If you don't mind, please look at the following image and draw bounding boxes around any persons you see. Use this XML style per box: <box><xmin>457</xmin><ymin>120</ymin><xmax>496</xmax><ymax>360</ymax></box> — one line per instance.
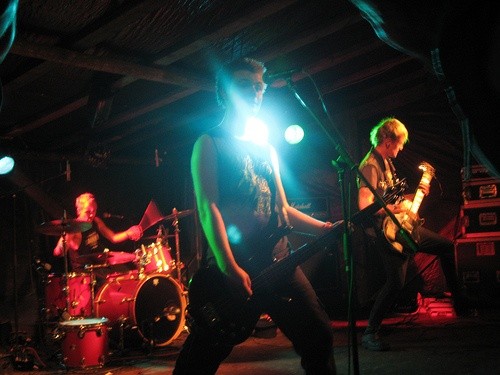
<box><xmin>357</xmin><ymin>119</ymin><xmax>477</xmax><ymax>351</ymax></box>
<box><xmin>54</xmin><ymin>193</ymin><xmax>143</xmax><ymax>294</ymax></box>
<box><xmin>172</xmin><ymin>58</ymin><xmax>352</xmax><ymax>375</ymax></box>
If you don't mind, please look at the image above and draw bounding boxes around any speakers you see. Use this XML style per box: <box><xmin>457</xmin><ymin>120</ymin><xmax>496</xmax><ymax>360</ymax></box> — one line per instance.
<box><xmin>453</xmin><ymin>239</ymin><xmax>500</xmax><ymax>319</ymax></box>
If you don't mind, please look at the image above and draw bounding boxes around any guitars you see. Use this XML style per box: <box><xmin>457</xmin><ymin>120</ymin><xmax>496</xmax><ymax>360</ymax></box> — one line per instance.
<box><xmin>186</xmin><ymin>172</ymin><xmax>407</xmax><ymax>346</ymax></box>
<box><xmin>382</xmin><ymin>160</ymin><xmax>437</xmax><ymax>257</ymax></box>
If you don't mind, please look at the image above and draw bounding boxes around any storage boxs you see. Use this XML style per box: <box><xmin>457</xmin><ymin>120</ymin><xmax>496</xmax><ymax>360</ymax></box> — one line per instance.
<box><xmin>454</xmin><ymin>165</ymin><xmax>500</xmax><ymax>317</ymax></box>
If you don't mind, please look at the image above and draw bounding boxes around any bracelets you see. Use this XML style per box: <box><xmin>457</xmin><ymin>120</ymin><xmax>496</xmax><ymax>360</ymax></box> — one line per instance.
<box><xmin>321</xmin><ymin>222</ymin><xmax>331</xmax><ymax>234</ymax></box>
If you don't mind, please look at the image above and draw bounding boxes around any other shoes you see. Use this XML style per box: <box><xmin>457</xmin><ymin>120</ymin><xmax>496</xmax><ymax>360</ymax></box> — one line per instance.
<box><xmin>362</xmin><ymin>327</ymin><xmax>390</xmax><ymax>351</ymax></box>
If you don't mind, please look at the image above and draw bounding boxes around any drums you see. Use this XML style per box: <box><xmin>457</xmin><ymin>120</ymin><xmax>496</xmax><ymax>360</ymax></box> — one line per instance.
<box><xmin>57</xmin><ymin>316</ymin><xmax>111</xmax><ymax>371</ymax></box>
<box><xmin>43</xmin><ymin>272</ymin><xmax>97</xmax><ymax>322</ymax></box>
<box><xmin>93</xmin><ymin>272</ymin><xmax>188</xmax><ymax>349</ymax></box>
<box><xmin>132</xmin><ymin>242</ymin><xmax>178</xmax><ymax>274</ymax></box>
<box><xmin>133</xmin><ymin>241</ymin><xmax>178</xmax><ymax>278</ymax></box>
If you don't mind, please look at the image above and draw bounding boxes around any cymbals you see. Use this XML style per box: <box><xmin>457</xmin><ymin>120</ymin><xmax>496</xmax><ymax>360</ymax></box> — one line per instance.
<box><xmin>34</xmin><ymin>218</ymin><xmax>93</xmax><ymax>236</ymax></box>
<box><xmin>139</xmin><ymin>234</ymin><xmax>176</xmax><ymax>240</ymax></box>
<box><xmin>69</xmin><ymin>251</ymin><xmax>136</xmax><ymax>266</ymax></box>
<box><xmin>159</xmin><ymin>208</ymin><xmax>195</xmax><ymax>220</ymax></box>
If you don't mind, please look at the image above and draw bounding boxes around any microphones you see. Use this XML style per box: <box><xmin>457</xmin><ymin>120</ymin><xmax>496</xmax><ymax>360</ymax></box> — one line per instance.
<box><xmin>263</xmin><ymin>67</ymin><xmax>302</xmax><ymax>83</ymax></box>
